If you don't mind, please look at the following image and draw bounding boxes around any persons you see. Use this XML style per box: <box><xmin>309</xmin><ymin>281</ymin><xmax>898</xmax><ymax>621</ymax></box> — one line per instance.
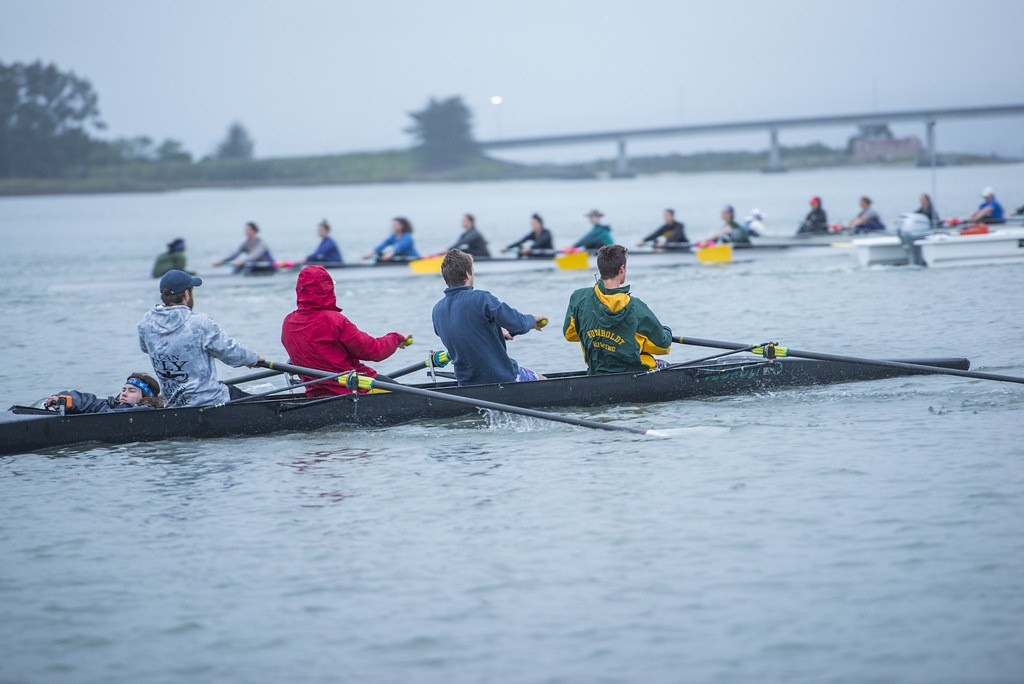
<box><xmin>712</xmin><ymin>206</ymin><xmax>750</xmax><ymax>242</ymax></box>
<box><xmin>432</xmin><ymin>248</ymin><xmax>547</xmax><ymax>386</ymax></box>
<box><xmin>971</xmin><ymin>189</ymin><xmax>1005</xmax><ymax>223</ymax></box>
<box><xmin>213</xmin><ymin>223</ymin><xmax>277</xmax><ymax>273</ymax></box>
<box><xmin>44</xmin><ymin>373</ymin><xmax>160</xmax><ymax>414</ymax></box>
<box><xmin>138</xmin><ymin>270</ymin><xmax>266</xmax><ymax>408</ymax></box>
<box><xmin>744</xmin><ymin>209</ymin><xmax>775</xmax><ymax>237</ymax></box>
<box><xmin>848</xmin><ymin>197</ymin><xmax>886</xmax><ymax>230</ymax></box>
<box><xmin>303</xmin><ymin>222</ymin><xmax>342</xmax><ymax>262</ymax></box>
<box><xmin>281</xmin><ymin>265</ymin><xmax>412</xmax><ymax>397</ymax></box>
<box><xmin>640</xmin><ymin>209</ymin><xmax>690</xmax><ymax>250</ymax></box>
<box><xmin>563</xmin><ymin>244</ymin><xmax>672</xmax><ymax>376</ymax></box>
<box><xmin>917</xmin><ymin>194</ymin><xmax>939</xmax><ymax>220</ymax></box>
<box><xmin>448</xmin><ymin>214</ymin><xmax>489</xmax><ymax>256</ymax></box>
<box><xmin>152</xmin><ymin>239</ymin><xmax>187</xmax><ymax>279</ymax></box>
<box><xmin>362</xmin><ymin>217</ymin><xmax>419</xmax><ymax>262</ymax></box>
<box><xmin>574</xmin><ymin>210</ymin><xmax>613</xmax><ymax>248</ymax></box>
<box><xmin>799</xmin><ymin>198</ymin><xmax>827</xmax><ymax>232</ymax></box>
<box><xmin>501</xmin><ymin>214</ymin><xmax>556</xmax><ymax>257</ymax></box>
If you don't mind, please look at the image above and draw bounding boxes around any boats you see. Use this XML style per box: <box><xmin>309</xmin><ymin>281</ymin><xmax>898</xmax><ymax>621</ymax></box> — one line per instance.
<box><xmin>0</xmin><ymin>345</ymin><xmax>972</xmax><ymax>461</ymax></box>
<box><xmin>44</xmin><ymin>211</ymin><xmax>1024</xmax><ymax>299</ymax></box>
<box><xmin>828</xmin><ymin>219</ymin><xmax>1024</xmax><ymax>272</ymax></box>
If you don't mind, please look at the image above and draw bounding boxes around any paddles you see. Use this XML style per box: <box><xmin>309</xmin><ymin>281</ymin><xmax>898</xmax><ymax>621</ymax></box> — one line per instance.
<box><xmin>389</xmin><ymin>317</ymin><xmax>547</xmax><ymax>380</ymax></box>
<box><xmin>224</xmin><ymin>253</ymin><xmax>445</xmax><ymax>276</ymax></box>
<box><xmin>223</xmin><ymin>337</ymin><xmax>413</xmax><ymax>385</ymax></box>
<box><xmin>671</xmin><ymin>334</ymin><xmax>1024</xmax><ymax>385</ymax></box>
<box><xmin>257</xmin><ymin>358</ymin><xmax>730</xmax><ymax>440</ymax></box>
<box><xmin>364</xmin><ymin>251</ymin><xmax>590</xmax><ymax>271</ymax></box>
<box><xmin>521</xmin><ymin>243</ymin><xmax>734</xmax><ymax>264</ymax></box>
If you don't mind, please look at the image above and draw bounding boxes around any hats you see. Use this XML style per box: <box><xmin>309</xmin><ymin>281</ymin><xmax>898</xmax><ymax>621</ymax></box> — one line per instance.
<box><xmin>977</xmin><ymin>187</ymin><xmax>994</xmax><ymax>197</ymax></box>
<box><xmin>583</xmin><ymin>209</ymin><xmax>604</xmax><ymax>217</ymax></box>
<box><xmin>160</xmin><ymin>270</ymin><xmax>202</xmax><ymax>295</ymax></box>
